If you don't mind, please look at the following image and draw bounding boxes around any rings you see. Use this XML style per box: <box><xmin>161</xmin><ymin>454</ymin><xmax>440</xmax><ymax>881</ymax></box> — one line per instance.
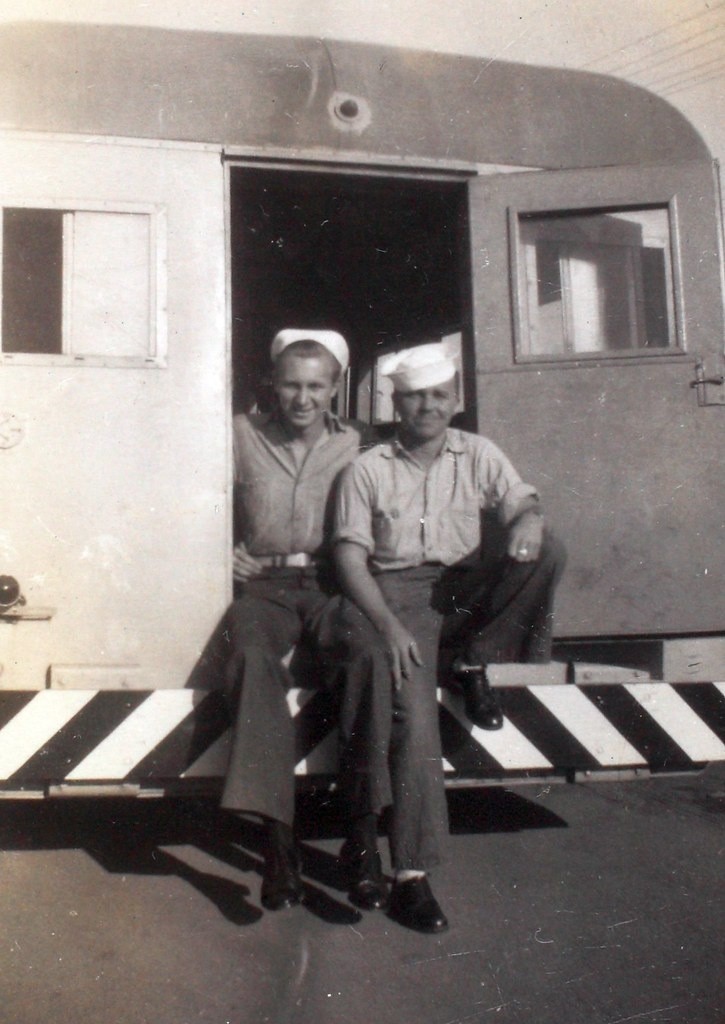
<box><xmin>516</xmin><ymin>548</ymin><xmax>528</xmax><ymax>557</ymax></box>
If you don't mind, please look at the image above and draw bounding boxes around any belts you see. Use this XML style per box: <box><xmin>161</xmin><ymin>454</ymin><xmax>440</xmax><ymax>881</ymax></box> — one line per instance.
<box><xmin>256</xmin><ymin>553</ymin><xmax>323</xmax><ymax>568</ymax></box>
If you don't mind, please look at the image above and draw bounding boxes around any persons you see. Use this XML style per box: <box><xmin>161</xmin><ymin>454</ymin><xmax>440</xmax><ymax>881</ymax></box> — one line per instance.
<box><xmin>330</xmin><ymin>341</ymin><xmax>566</xmax><ymax>933</ymax></box>
<box><xmin>210</xmin><ymin>325</ymin><xmax>397</xmax><ymax>911</ymax></box>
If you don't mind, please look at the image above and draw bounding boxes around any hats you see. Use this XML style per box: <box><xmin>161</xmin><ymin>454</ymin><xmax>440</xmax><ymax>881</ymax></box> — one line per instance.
<box><xmin>378</xmin><ymin>342</ymin><xmax>456</xmax><ymax>392</ymax></box>
<box><xmin>270</xmin><ymin>329</ymin><xmax>350</xmax><ymax>372</ymax></box>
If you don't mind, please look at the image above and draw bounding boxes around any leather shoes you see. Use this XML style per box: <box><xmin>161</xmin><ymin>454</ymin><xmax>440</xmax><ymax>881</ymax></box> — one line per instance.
<box><xmin>443</xmin><ymin>660</ymin><xmax>504</xmax><ymax>730</ymax></box>
<box><xmin>261</xmin><ymin>840</ymin><xmax>303</xmax><ymax>909</ymax></box>
<box><xmin>336</xmin><ymin>843</ymin><xmax>387</xmax><ymax>910</ymax></box>
<box><xmin>385</xmin><ymin>873</ymin><xmax>448</xmax><ymax>933</ymax></box>
